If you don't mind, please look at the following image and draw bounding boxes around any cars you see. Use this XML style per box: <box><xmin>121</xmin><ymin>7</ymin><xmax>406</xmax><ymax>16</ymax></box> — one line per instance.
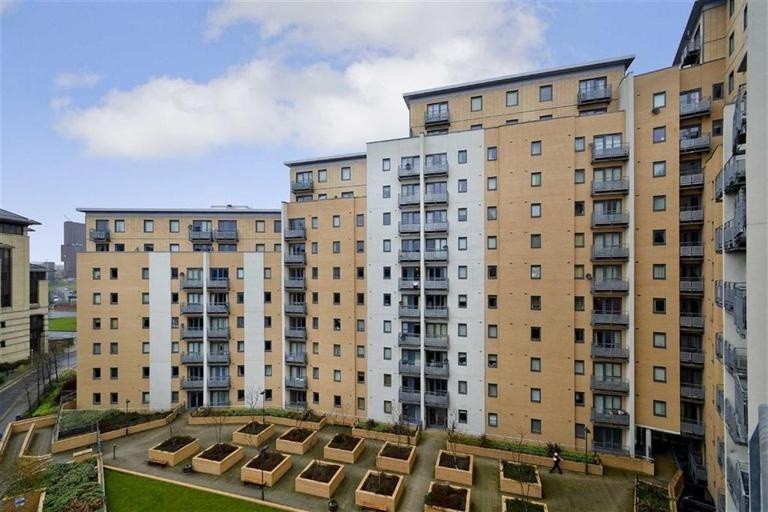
<box><xmin>53</xmin><ymin>289</ymin><xmax>76</xmax><ymax>304</ymax></box>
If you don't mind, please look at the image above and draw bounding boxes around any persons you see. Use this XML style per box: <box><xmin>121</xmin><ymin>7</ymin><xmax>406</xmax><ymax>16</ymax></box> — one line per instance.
<box><xmin>549</xmin><ymin>449</ymin><xmax>563</xmax><ymax>474</ymax></box>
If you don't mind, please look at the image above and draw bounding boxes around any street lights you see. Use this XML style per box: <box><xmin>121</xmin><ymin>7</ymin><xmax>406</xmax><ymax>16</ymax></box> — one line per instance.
<box><xmin>584</xmin><ymin>426</ymin><xmax>591</xmax><ymax>473</ymax></box>
<box><xmin>260</xmin><ymin>445</ymin><xmax>270</xmax><ymax>500</ymax></box>
<box><xmin>260</xmin><ymin>391</ymin><xmax>265</xmax><ymax>423</ymax></box>
<box><xmin>126</xmin><ymin>398</ymin><xmax>131</xmax><ymax>436</ymax></box>
